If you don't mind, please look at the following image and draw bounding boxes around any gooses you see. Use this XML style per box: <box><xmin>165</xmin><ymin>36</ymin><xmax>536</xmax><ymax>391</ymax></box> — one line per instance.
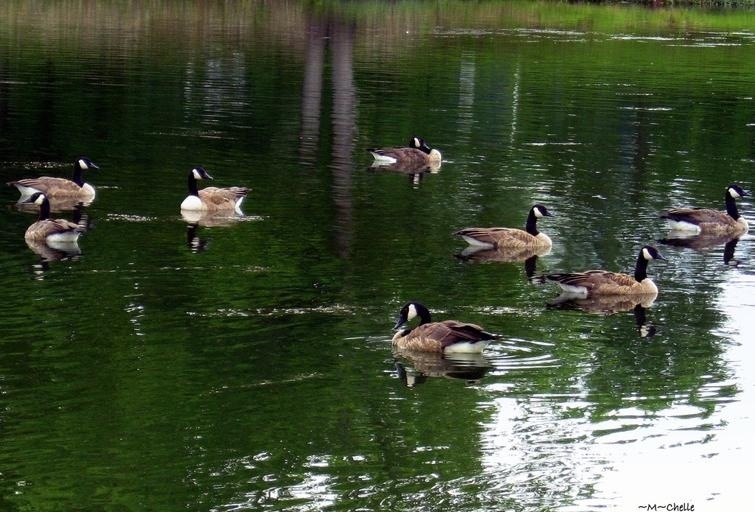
<box><xmin>550</xmin><ymin>247</ymin><xmax>670</xmax><ymax>297</ymax></box>
<box><xmin>451</xmin><ymin>203</ymin><xmax>557</xmax><ymax>249</ymax></box>
<box><xmin>392</xmin><ymin>347</ymin><xmax>496</xmax><ymax>388</ymax></box>
<box><xmin>546</xmin><ymin>291</ymin><xmax>660</xmax><ymax>340</ymax></box>
<box><xmin>383</xmin><ymin>303</ymin><xmax>504</xmax><ymax>356</ymax></box>
<box><xmin>6</xmin><ymin>157</ymin><xmax>99</xmax><ymax>243</ymax></box>
<box><xmin>659</xmin><ymin>185</ymin><xmax>753</xmax><ymax>232</ymax></box>
<box><xmin>454</xmin><ymin>244</ymin><xmax>552</xmax><ymax>288</ymax></box>
<box><xmin>180</xmin><ymin>209</ymin><xmax>264</xmax><ymax>251</ymax></box>
<box><xmin>180</xmin><ymin>167</ymin><xmax>253</xmax><ymax>209</ymax></box>
<box><xmin>365</xmin><ymin>136</ymin><xmax>441</xmax><ymax>163</ymax></box>
<box><xmin>655</xmin><ymin>230</ymin><xmax>747</xmax><ymax>268</ymax></box>
